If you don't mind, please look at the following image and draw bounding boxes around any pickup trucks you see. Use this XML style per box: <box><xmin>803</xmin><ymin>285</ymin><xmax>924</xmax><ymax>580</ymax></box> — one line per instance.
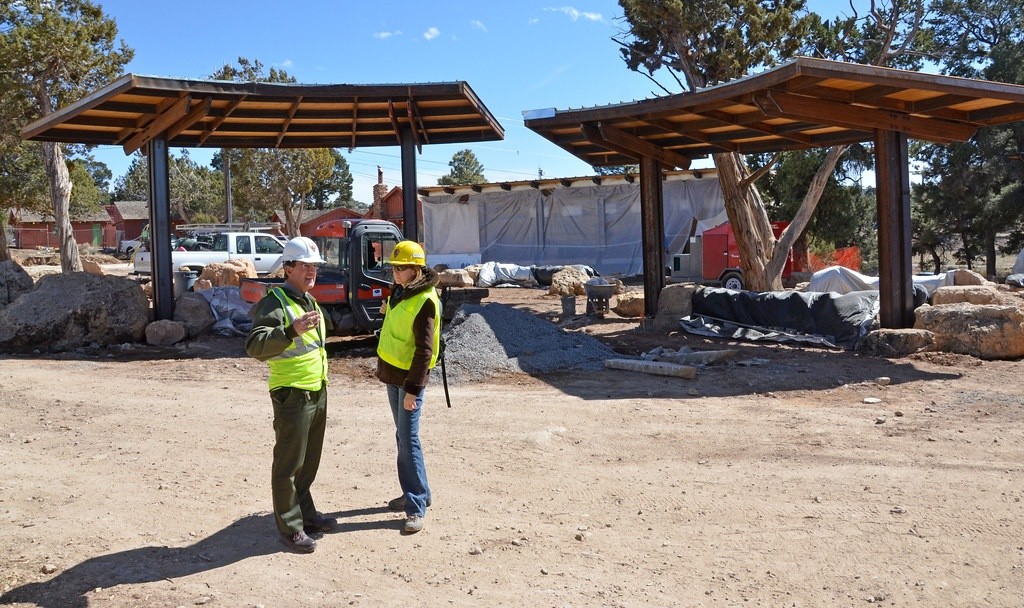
<box><xmin>128</xmin><ymin>233</ymin><xmax>285</xmax><ymax>278</ymax></box>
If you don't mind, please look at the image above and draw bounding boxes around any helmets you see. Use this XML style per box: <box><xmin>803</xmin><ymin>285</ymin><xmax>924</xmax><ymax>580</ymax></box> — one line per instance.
<box><xmin>281</xmin><ymin>237</ymin><xmax>326</xmax><ymax>263</ymax></box>
<box><xmin>385</xmin><ymin>241</ymin><xmax>426</xmax><ymax>266</ymax></box>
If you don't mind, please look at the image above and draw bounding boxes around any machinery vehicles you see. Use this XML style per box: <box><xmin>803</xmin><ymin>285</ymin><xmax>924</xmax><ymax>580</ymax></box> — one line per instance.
<box><xmin>239</xmin><ymin>219</ymin><xmax>404</xmax><ymax>336</ymax></box>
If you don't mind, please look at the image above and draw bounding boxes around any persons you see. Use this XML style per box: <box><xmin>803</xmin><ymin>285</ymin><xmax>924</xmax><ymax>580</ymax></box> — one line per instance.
<box><xmin>375</xmin><ymin>239</ymin><xmax>441</xmax><ymax>533</ymax></box>
<box><xmin>245</xmin><ymin>238</ymin><xmax>337</xmax><ymax>553</ymax></box>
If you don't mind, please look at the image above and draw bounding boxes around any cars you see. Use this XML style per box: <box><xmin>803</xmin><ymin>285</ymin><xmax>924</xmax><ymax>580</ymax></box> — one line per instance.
<box><xmin>119</xmin><ymin>234</ymin><xmax>177</xmax><ymax>260</ymax></box>
<box><xmin>263</xmin><ymin>236</ymin><xmax>289</xmax><ymax>248</ymax></box>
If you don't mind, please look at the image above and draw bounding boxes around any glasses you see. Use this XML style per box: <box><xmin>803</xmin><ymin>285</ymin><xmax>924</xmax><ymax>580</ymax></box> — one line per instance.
<box><xmin>293</xmin><ymin>262</ymin><xmax>318</xmax><ymax>270</ymax></box>
<box><xmin>390</xmin><ymin>265</ymin><xmax>419</xmax><ymax>271</ymax></box>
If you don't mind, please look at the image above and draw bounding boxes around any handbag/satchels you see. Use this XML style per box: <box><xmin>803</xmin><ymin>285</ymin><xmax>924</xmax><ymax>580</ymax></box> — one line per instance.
<box><xmin>436</xmin><ymin>299</ymin><xmax>452</xmax><ymax>408</ymax></box>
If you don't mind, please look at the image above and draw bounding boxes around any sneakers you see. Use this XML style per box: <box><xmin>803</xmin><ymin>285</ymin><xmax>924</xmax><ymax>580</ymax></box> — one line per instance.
<box><xmin>389</xmin><ymin>494</ymin><xmax>431</xmax><ymax>510</ymax></box>
<box><xmin>404</xmin><ymin>516</ymin><xmax>423</xmax><ymax>531</ymax></box>
<box><xmin>278</xmin><ymin>530</ymin><xmax>316</xmax><ymax>554</ymax></box>
<box><xmin>303</xmin><ymin>515</ymin><xmax>337</xmax><ymax>534</ymax></box>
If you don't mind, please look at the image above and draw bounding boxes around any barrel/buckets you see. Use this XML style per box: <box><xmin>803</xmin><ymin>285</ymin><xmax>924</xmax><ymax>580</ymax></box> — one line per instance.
<box><xmin>561</xmin><ymin>295</ymin><xmax>575</xmax><ymax>314</ymax></box>
<box><xmin>174</xmin><ymin>271</ymin><xmax>197</xmax><ymax>299</ymax></box>
